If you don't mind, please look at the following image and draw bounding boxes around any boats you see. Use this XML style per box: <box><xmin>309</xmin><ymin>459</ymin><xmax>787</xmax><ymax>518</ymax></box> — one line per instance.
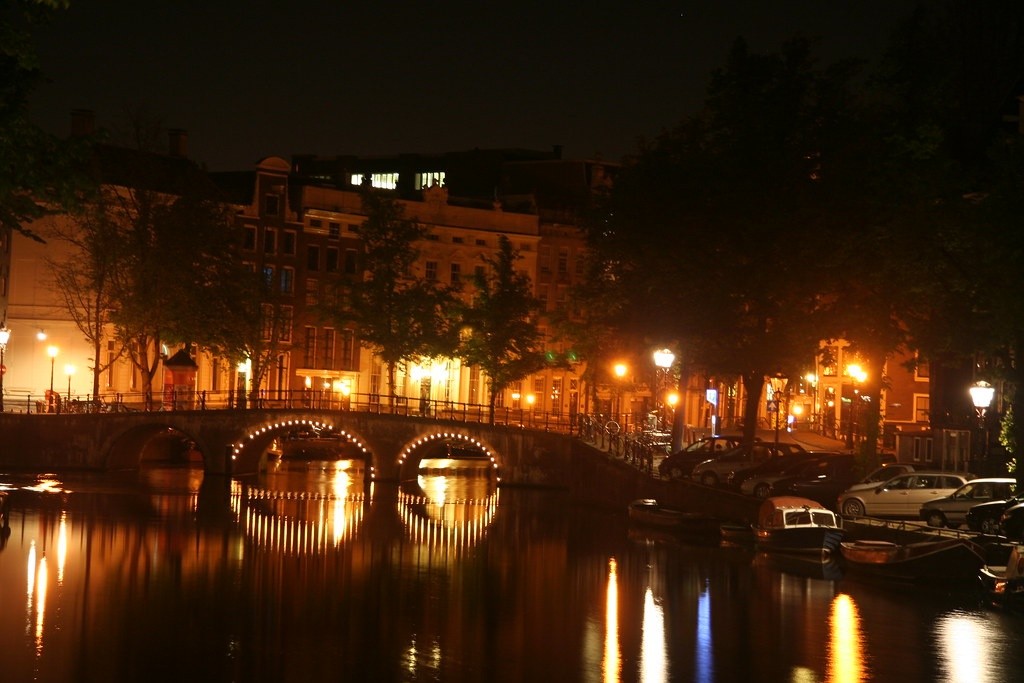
<box><xmin>626</xmin><ymin>496</ymin><xmax>848</xmax><ymax>555</ymax></box>
<box><xmin>839</xmin><ymin>537</ymin><xmax>990</xmax><ymax>581</ymax></box>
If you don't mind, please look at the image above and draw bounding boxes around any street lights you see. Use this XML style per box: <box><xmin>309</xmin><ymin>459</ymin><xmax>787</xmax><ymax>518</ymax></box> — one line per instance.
<box><xmin>968</xmin><ymin>380</ymin><xmax>995</xmax><ymax>478</ymax></box>
<box><xmin>48</xmin><ymin>345</ymin><xmax>59</xmax><ymax>414</ymax></box>
<box><xmin>66</xmin><ymin>365</ymin><xmax>76</xmax><ymax>400</ymax></box>
<box><xmin>239</xmin><ymin>363</ymin><xmax>246</xmax><ymax>390</ymax></box>
<box><xmin>0</xmin><ymin>321</ymin><xmax>11</xmax><ymax>412</ymax></box>
<box><xmin>654</xmin><ymin>349</ymin><xmax>675</xmax><ymax>433</ymax></box>
<box><xmin>770</xmin><ymin>364</ymin><xmax>867</xmax><ymax>450</ymax></box>
<box><xmin>615</xmin><ymin>365</ymin><xmax>626</xmax><ymax>456</ymax></box>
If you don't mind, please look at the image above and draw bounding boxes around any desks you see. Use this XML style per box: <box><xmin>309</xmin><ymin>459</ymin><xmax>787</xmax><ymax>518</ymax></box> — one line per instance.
<box><xmin>635</xmin><ymin>430</ymin><xmax>671</xmax><ymax>454</ymax></box>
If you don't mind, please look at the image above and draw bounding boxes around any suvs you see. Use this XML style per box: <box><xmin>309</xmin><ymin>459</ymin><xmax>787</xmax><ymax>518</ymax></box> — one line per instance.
<box><xmin>659</xmin><ymin>434</ymin><xmax>978</xmax><ymax>518</ymax></box>
<box><xmin>966</xmin><ymin>491</ymin><xmax>1024</xmax><ymax>539</ymax></box>
<box><xmin>920</xmin><ymin>478</ymin><xmax>1017</xmax><ymax>529</ymax></box>
